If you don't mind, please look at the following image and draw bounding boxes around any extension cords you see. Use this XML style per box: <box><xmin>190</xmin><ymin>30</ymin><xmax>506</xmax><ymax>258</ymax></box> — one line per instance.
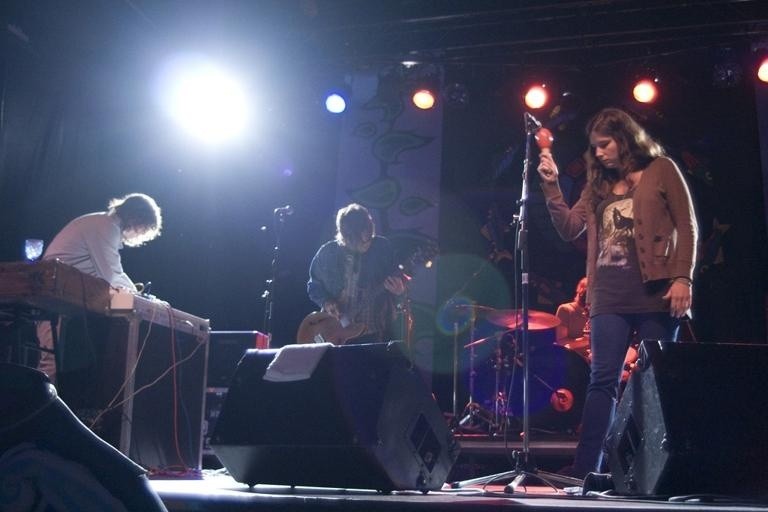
<box><xmin>135</xmin><ymin>296</ymin><xmax>171</xmax><ymax>307</ymax></box>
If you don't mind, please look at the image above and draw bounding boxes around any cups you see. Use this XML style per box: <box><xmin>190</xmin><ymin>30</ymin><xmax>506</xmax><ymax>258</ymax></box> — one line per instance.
<box><xmin>25</xmin><ymin>238</ymin><xmax>44</xmax><ymax>264</ymax></box>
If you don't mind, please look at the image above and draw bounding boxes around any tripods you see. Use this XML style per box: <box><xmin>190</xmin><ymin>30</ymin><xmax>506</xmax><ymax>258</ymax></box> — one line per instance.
<box><xmin>444</xmin><ymin>297</ymin><xmax>500</xmax><ymax>438</ymax></box>
<box><xmin>451</xmin><ymin>114</ymin><xmax>585</xmax><ymax>497</ymax></box>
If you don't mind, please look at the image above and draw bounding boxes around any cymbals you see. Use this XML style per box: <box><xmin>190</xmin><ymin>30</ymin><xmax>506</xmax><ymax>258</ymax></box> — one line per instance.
<box><xmin>486</xmin><ymin>308</ymin><xmax>561</xmax><ymax>331</ymax></box>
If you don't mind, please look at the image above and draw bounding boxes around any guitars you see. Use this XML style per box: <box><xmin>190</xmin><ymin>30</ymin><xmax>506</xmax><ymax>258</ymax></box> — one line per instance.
<box><xmin>296</xmin><ymin>238</ymin><xmax>442</xmax><ymax>345</ymax></box>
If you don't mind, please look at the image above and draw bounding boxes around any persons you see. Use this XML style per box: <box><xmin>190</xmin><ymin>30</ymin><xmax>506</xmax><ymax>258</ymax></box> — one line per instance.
<box><xmin>30</xmin><ymin>193</ymin><xmax>163</xmax><ymax>385</ymax></box>
<box><xmin>536</xmin><ymin>106</ymin><xmax>701</xmax><ymax>490</ymax></box>
<box><xmin>306</xmin><ymin>203</ymin><xmax>409</xmax><ymax>346</ymax></box>
<box><xmin>554</xmin><ymin>277</ymin><xmax>639</xmax><ymax>381</ymax></box>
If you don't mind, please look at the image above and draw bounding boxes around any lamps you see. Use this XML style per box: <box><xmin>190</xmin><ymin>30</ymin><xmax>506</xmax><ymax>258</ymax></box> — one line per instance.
<box><xmin>323</xmin><ymin>87</ymin><xmax>346</xmax><ymax>116</ymax></box>
<box><xmin>410</xmin><ymin>82</ymin><xmax>440</xmax><ymax>110</ymax></box>
<box><xmin>755</xmin><ymin>55</ymin><xmax>768</xmax><ymax>84</ymax></box>
<box><xmin>520</xmin><ymin>79</ymin><xmax>553</xmax><ymax>109</ymax></box>
<box><xmin>632</xmin><ymin>73</ymin><xmax>661</xmax><ymax>104</ymax></box>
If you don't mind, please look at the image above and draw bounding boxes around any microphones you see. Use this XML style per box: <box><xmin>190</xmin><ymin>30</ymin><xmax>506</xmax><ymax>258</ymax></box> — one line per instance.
<box><xmin>536</xmin><ymin>128</ymin><xmax>556</xmax><ymax>179</ymax></box>
<box><xmin>271</xmin><ymin>205</ymin><xmax>295</xmax><ymax>217</ymax></box>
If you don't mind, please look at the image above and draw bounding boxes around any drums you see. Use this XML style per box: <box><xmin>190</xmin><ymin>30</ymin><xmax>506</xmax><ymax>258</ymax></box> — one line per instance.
<box><xmin>506</xmin><ymin>344</ymin><xmax>591</xmax><ymax>427</ymax></box>
